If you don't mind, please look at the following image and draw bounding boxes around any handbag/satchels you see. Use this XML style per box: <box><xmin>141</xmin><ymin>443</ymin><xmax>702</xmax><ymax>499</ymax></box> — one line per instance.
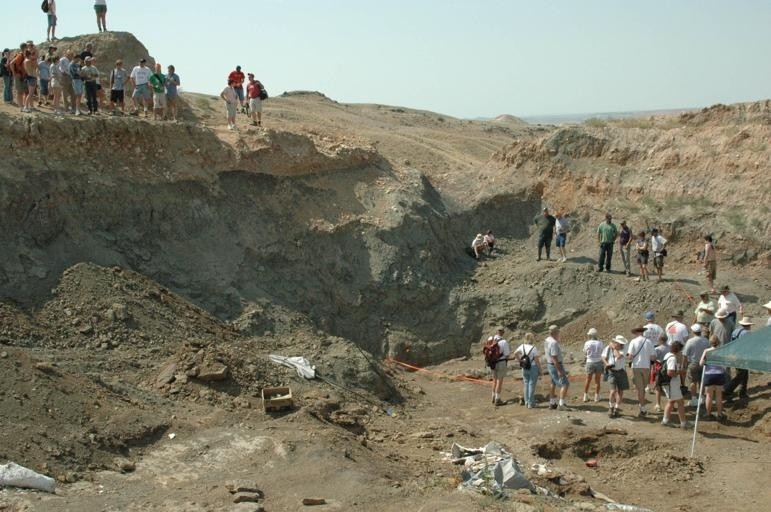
<box><xmin>256</xmin><ymin>80</ymin><xmax>269</xmax><ymax>100</ymax></box>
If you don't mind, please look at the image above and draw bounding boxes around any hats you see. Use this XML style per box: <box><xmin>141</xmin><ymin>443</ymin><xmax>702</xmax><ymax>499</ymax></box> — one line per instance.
<box><xmin>761</xmin><ymin>301</ymin><xmax>770</xmax><ymax>308</ymax></box>
<box><xmin>587</xmin><ymin>327</ymin><xmax>597</xmax><ymax>335</ymax></box>
<box><xmin>631</xmin><ymin>286</ymin><xmax>755</xmax><ymax>347</ymax></box>
<box><xmin>612</xmin><ymin>335</ymin><xmax>628</xmax><ymax>345</ymax></box>
<box><xmin>476</xmin><ymin>233</ymin><xmax>483</xmax><ymax>240</ymax></box>
<box><xmin>619</xmin><ymin>220</ymin><xmax>626</xmax><ymax>224</ymax></box>
<box><xmin>548</xmin><ymin>325</ymin><xmax>559</xmax><ymax>333</ymax></box>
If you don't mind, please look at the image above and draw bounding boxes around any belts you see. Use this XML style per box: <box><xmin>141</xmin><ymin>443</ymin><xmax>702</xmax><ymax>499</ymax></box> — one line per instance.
<box><xmin>551</xmin><ymin>361</ymin><xmax>561</xmax><ymax>365</ymax></box>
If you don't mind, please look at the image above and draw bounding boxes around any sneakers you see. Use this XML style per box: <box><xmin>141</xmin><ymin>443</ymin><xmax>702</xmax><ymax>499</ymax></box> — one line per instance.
<box><xmin>496</xmin><ymin>399</ymin><xmax>507</xmax><ymax>405</ymax></box>
<box><xmin>608</xmin><ymin>389</ymin><xmax>748</xmax><ymax>428</ymax></box>
<box><xmin>595</xmin><ymin>394</ymin><xmax>601</xmax><ymax>403</ymax></box>
<box><xmin>584</xmin><ymin>394</ymin><xmax>590</xmax><ymax>402</ymax></box>
<box><xmin>557</xmin><ymin>404</ymin><xmax>571</xmax><ymax>410</ymax></box>
<box><xmin>529</xmin><ymin>403</ymin><xmax>538</xmax><ymax>409</ymax></box>
<box><xmin>549</xmin><ymin>403</ymin><xmax>557</xmax><ymax>408</ymax></box>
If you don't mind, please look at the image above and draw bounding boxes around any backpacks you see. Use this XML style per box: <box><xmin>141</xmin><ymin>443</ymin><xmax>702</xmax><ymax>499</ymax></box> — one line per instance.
<box><xmin>521</xmin><ymin>345</ymin><xmax>536</xmax><ymax>369</ymax></box>
<box><xmin>653</xmin><ymin>360</ymin><xmax>671</xmax><ymax>386</ymax></box>
<box><xmin>483</xmin><ymin>336</ymin><xmax>503</xmax><ymax>368</ymax></box>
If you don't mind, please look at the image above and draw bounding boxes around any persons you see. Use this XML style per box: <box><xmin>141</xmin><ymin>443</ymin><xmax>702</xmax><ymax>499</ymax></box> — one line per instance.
<box><xmin>579</xmin><ymin>328</ymin><xmax>605</xmax><ymax>404</ymax></box>
<box><xmin>544</xmin><ymin>324</ymin><xmax>573</xmax><ymax>412</ymax></box>
<box><xmin>129</xmin><ymin>58</ymin><xmax>154</xmax><ymax>118</ymax></box>
<box><xmin>634</xmin><ymin>228</ymin><xmax>651</xmax><ymax>283</ymax></box>
<box><xmin>471</xmin><ymin>233</ymin><xmax>489</xmax><ymax>259</ymax></box>
<box><xmin>553</xmin><ymin>211</ymin><xmax>571</xmax><ymax>264</ymax></box>
<box><xmin>650</xmin><ymin>227</ymin><xmax>669</xmax><ymax>283</ymax></box>
<box><xmin>92</xmin><ymin>0</ymin><xmax>108</xmax><ymax>33</ymax></box>
<box><xmin>601</xmin><ymin>333</ymin><xmax>630</xmax><ymax>419</ymax></box>
<box><xmin>107</xmin><ymin>59</ymin><xmax>128</xmax><ymax>115</ymax></box>
<box><xmin>1</xmin><ymin>36</ymin><xmax>100</xmax><ymax>133</ymax></box>
<box><xmin>484</xmin><ymin>325</ymin><xmax>512</xmax><ymax>405</ymax></box>
<box><xmin>245</xmin><ymin>72</ymin><xmax>267</xmax><ymax>128</ymax></box>
<box><xmin>618</xmin><ymin>219</ymin><xmax>633</xmax><ymax>277</ymax></box>
<box><xmin>162</xmin><ymin>63</ymin><xmax>180</xmax><ymax>123</ymax></box>
<box><xmin>483</xmin><ymin>229</ymin><xmax>496</xmax><ymax>257</ymax></box>
<box><xmin>595</xmin><ymin>212</ymin><xmax>617</xmax><ymax>273</ymax></box>
<box><xmin>641</xmin><ymin>283</ymin><xmax>771</xmax><ymax>431</ymax></box>
<box><xmin>701</xmin><ymin>234</ymin><xmax>720</xmax><ymax>295</ymax></box>
<box><xmin>627</xmin><ymin>323</ymin><xmax>657</xmax><ymax>419</ymax></box>
<box><xmin>513</xmin><ymin>333</ymin><xmax>544</xmax><ymax>409</ymax></box>
<box><xmin>218</xmin><ymin>78</ymin><xmax>239</xmax><ymax>130</ymax></box>
<box><xmin>532</xmin><ymin>208</ymin><xmax>556</xmax><ymax>262</ymax></box>
<box><xmin>146</xmin><ymin>62</ymin><xmax>166</xmax><ymax>121</ymax></box>
<box><xmin>228</xmin><ymin>64</ymin><xmax>246</xmax><ymax>114</ymax></box>
<box><xmin>40</xmin><ymin>0</ymin><xmax>57</xmax><ymax>42</ymax></box>
